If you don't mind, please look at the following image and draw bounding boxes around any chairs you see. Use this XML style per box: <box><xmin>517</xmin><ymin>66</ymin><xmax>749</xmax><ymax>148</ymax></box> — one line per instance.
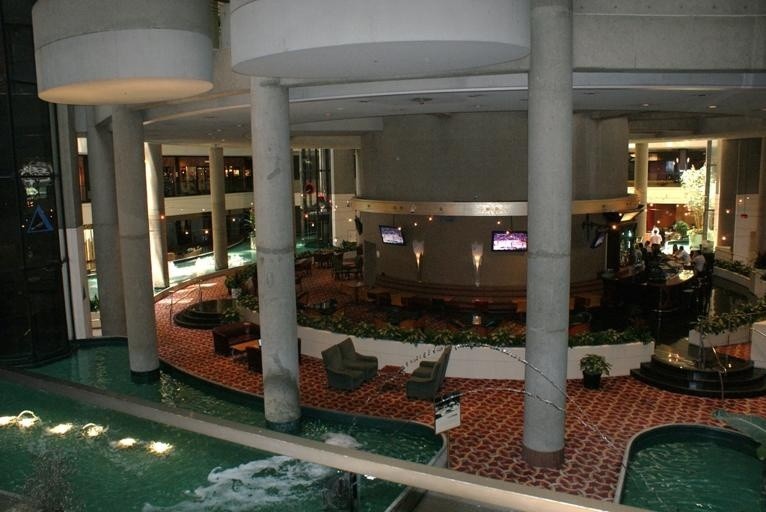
<box><xmin>295</xmin><ymin>253</ymin><xmax>335</xmax><ymax>291</ymax></box>
<box><xmin>321</xmin><ymin>337</ymin><xmax>378</xmax><ymax>392</ymax></box>
<box><xmin>406</xmin><ymin>345</ymin><xmax>452</xmax><ymax>402</ymax></box>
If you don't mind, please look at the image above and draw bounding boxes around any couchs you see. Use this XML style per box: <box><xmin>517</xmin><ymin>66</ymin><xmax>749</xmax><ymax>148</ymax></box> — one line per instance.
<box><xmin>212</xmin><ymin>321</ymin><xmax>301</xmax><ymax>375</ymax></box>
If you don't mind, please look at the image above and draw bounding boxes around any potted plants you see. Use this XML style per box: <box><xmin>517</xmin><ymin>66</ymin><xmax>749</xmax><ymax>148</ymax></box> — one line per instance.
<box><xmin>580</xmin><ymin>353</ymin><xmax>613</xmax><ymax>389</ymax></box>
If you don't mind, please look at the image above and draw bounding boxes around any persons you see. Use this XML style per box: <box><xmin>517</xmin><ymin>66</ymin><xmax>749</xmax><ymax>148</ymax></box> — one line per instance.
<box><xmin>633</xmin><ymin>229</ymin><xmax>706</xmax><ymax>279</ymax></box>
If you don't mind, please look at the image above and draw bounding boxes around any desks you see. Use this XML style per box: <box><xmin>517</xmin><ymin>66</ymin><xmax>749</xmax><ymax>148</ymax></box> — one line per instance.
<box><xmin>296</xmin><ymin>289</ymin><xmax>308</xmax><ymax>305</ymax></box>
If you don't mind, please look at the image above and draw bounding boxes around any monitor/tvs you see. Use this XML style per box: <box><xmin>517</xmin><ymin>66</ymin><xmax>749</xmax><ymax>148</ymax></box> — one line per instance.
<box><xmin>491</xmin><ymin>231</ymin><xmax>527</xmax><ymax>252</ymax></box>
<box><xmin>378</xmin><ymin>225</ymin><xmax>406</xmax><ymax>247</ymax></box>
<box><xmin>590</xmin><ymin>228</ymin><xmax>609</xmax><ymax>249</ymax></box>
